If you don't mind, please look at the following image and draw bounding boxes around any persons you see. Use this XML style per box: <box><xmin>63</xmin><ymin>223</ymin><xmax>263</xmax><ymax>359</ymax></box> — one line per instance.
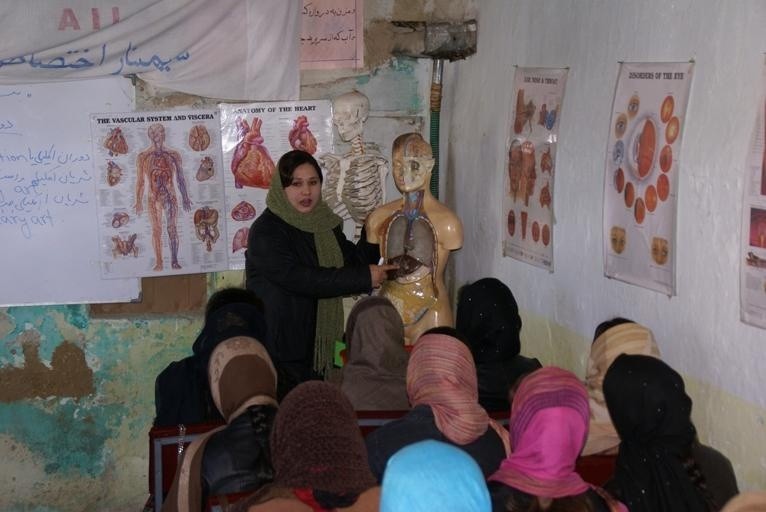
<box><xmin>153</xmin><ymin>287</ymin><xmax>509</xmax><ymax>512</ymax></box>
<box><xmin>457</xmin><ymin>278</ymin><xmax>766</xmax><ymax>512</ymax></box>
<box><xmin>361</xmin><ymin>132</ymin><xmax>464</xmax><ymax>347</ymax></box>
<box><xmin>244</xmin><ymin>150</ymin><xmax>400</xmax><ymax>381</ymax></box>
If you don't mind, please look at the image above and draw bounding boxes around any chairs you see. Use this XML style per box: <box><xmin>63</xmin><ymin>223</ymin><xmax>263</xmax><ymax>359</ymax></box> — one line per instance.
<box><xmin>147</xmin><ymin>377</ymin><xmax>765</xmax><ymax>511</ymax></box>
